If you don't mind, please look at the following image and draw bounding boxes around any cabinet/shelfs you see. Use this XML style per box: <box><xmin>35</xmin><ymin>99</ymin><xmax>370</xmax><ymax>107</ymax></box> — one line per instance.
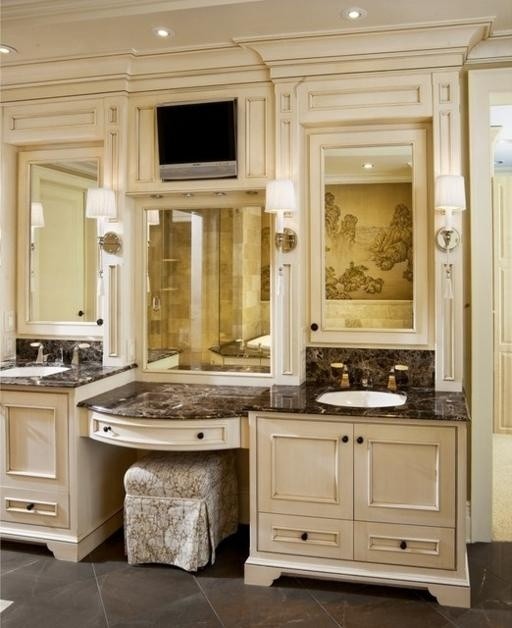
<box><xmin>1</xmin><ymin>363</ymin><xmax>137</xmax><ymax>562</ymax></box>
<box><xmin>80</xmin><ymin>389</ymin><xmax>267</xmax><ymax>451</ymax></box>
<box><xmin>244</xmin><ymin>384</ymin><xmax>473</xmax><ymax>610</ymax></box>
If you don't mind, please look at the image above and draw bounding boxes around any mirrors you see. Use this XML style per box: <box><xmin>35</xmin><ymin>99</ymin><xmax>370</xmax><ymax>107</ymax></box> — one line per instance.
<box><xmin>16</xmin><ymin>149</ymin><xmax>110</xmax><ymax>344</ymax></box>
<box><xmin>123</xmin><ymin>186</ymin><xmax>281</xmax><ymax>388</ymax></box>
<box><xmin>312</xmin><ymin>128</ymin><xmax>430</xmax><ymax>345</ymax></box>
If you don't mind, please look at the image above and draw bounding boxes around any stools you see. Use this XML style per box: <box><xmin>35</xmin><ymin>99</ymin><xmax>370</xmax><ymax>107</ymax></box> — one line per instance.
<box><xmin>121</xmin><ymin>453</ymin><xmax>237</xmax><ymax>571</ymax></box>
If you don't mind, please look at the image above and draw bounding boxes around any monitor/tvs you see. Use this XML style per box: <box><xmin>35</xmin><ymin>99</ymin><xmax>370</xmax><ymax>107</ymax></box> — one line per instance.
<box><xmin>155</xmin><ymin>97</ymin><xmax>238</xmax><ymax>181</ymax></box>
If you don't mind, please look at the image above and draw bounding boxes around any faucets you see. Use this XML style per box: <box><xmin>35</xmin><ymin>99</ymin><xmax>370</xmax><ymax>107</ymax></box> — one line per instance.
<box><xmin>359</xmin><ymin>367</ymin><xmax>373</xmax><ymax>388</ymax></box>
<box><xmin>256</xmin><ymin>341</ymin><xmax>263</xmax><ymax>352</ymax></box>
<box><xmin>53</xmin><ymin>345</ymin><xmax>66</xmax><ymax>364</ymax></box>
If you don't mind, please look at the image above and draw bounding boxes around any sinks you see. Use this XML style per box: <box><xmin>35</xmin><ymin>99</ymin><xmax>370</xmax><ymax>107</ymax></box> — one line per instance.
<box><xmin>315</xmin><ymin>390</ymin><xmax>408</xmax><ymax>409</ymax></box>
<box><xmin>0</xmin><ymin>364</ymin><xmax>72</xmax><ymax>379</ymax></box>
<box><xmin>245</xmin><ymin>333</ymin><xmax>270</xmax><ymax>352</ymax></box>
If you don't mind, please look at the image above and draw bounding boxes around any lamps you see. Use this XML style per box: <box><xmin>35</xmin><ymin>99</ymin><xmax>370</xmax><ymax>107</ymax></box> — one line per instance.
<box><xmin>429</xmin><ymin>174</ymin><xmax>466</xmax><ymax>252</ymax></box>
<box><xmin>84</xmin><ymin>187</ymin><xmax>121</xmax><ymax>254</ymax></box>
<box><xmin>264</xmin><ymin>178</ymin><xmax>299</xmax><ymax>253</ymax></box>
